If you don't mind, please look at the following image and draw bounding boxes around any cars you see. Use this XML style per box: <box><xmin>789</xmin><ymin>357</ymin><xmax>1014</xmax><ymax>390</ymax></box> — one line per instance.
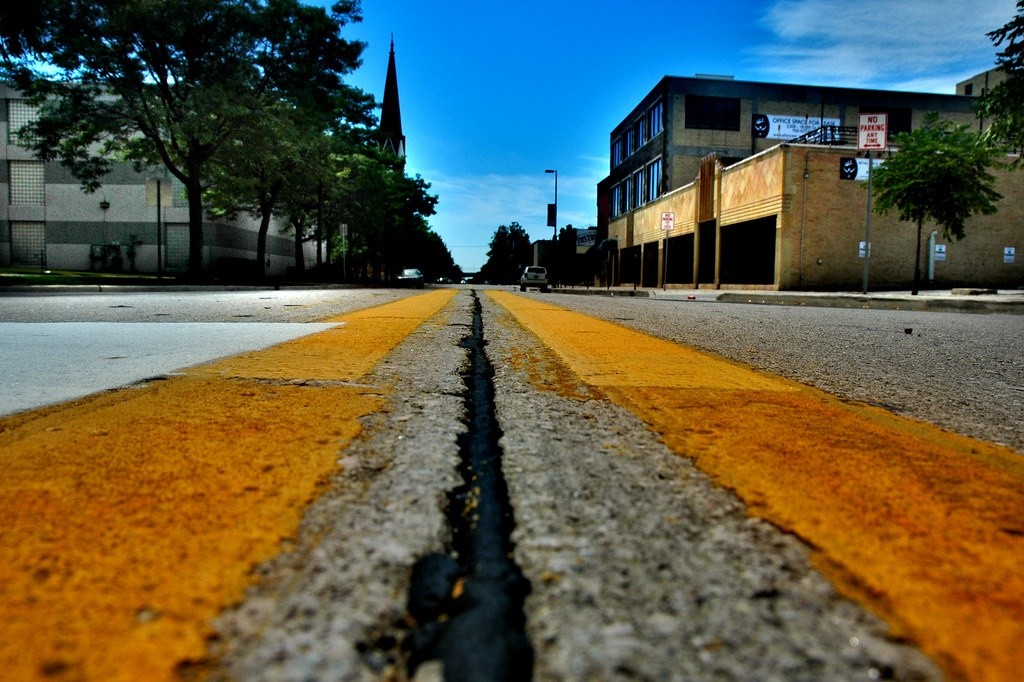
<box><xmin>461</xmin><ymin>280</ymin><xmax>466</xmax><ymax>284</ymax></box>
<box><xmin>396</xmin><ymin>269</ymin><xmax>424</xmax><ymax>289</ymax></box>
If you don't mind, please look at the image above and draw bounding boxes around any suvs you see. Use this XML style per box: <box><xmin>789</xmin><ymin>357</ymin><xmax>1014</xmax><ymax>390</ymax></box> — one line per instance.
<box><xmin>520</xmin><ymin>266</ymin><xmax>548</xmax><ymax>293</ymax></box>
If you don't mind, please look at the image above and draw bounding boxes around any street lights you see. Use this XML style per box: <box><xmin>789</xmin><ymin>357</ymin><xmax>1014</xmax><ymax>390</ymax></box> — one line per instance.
<box><xmin>545</xmin><ymin>170</ymin><xmax>557</xmax><ymax>240</ymax></box>
